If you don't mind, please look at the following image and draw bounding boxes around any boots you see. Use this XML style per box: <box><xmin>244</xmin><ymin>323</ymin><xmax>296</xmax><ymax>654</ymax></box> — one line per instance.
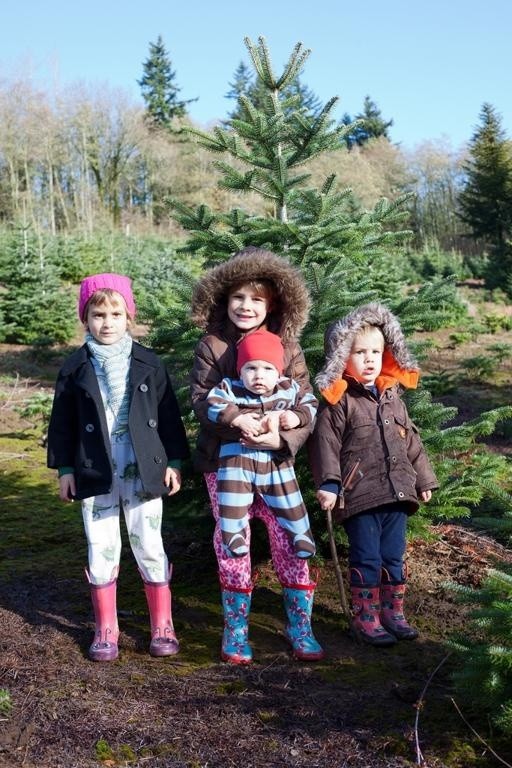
<box><xmin>218</xmin><ymin>581</ymin><xmax>255</xmax><ymax>666</ymax></box>
<box><xmin>143</xmin><ymin>578</ymin><xmax>184</xmax><ymax>654</ymax></box>
<box><xmin>381</xmin><ymin>582</ymin><xmax>420</xmax><ymax>642</ymax></box>
<box><xmin>86</xmin><ymin>578</ymin><xmax>121</xmax><ymax>661</ymax></box>
<box><xmin>350</xmin><ymin>580</ymin><xmax>401</xmax><ymax>645</ymax></box>
<box><xmin>280</xmin><ymin>583</ymin><xmax>326</xmax><ymax>661</ymax></box>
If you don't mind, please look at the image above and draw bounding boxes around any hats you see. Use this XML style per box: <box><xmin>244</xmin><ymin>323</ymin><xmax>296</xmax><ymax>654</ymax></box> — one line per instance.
<box><xmin>77</xmin><ymin>272</ymin><xmax>136</xmax><ymax>323</ymax></box>
<box><xmin>235</xmin><ymin>328</ymin><xmax>287</xmax><ymax>382</ymax></box>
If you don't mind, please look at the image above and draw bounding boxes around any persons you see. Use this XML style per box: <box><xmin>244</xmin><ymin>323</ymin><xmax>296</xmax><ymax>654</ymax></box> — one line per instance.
<box><xmin>203</xmin><ymin>327</ymin><xmax>321</xmax><ymax>559</ymax></box>
<box><xmin>305</xmin><ymin>301</ymin><xmax>443</xmax><ymax>645</ymax></box>
<box><xmin>44</xmin><ymin>269</ymin><xmax>194</xmax><ymax>660</ymax></box>
<box><xmin>188</xmin><ymin>246</ymin><xmax>327</xmax><ymax>664</ymax></box>
